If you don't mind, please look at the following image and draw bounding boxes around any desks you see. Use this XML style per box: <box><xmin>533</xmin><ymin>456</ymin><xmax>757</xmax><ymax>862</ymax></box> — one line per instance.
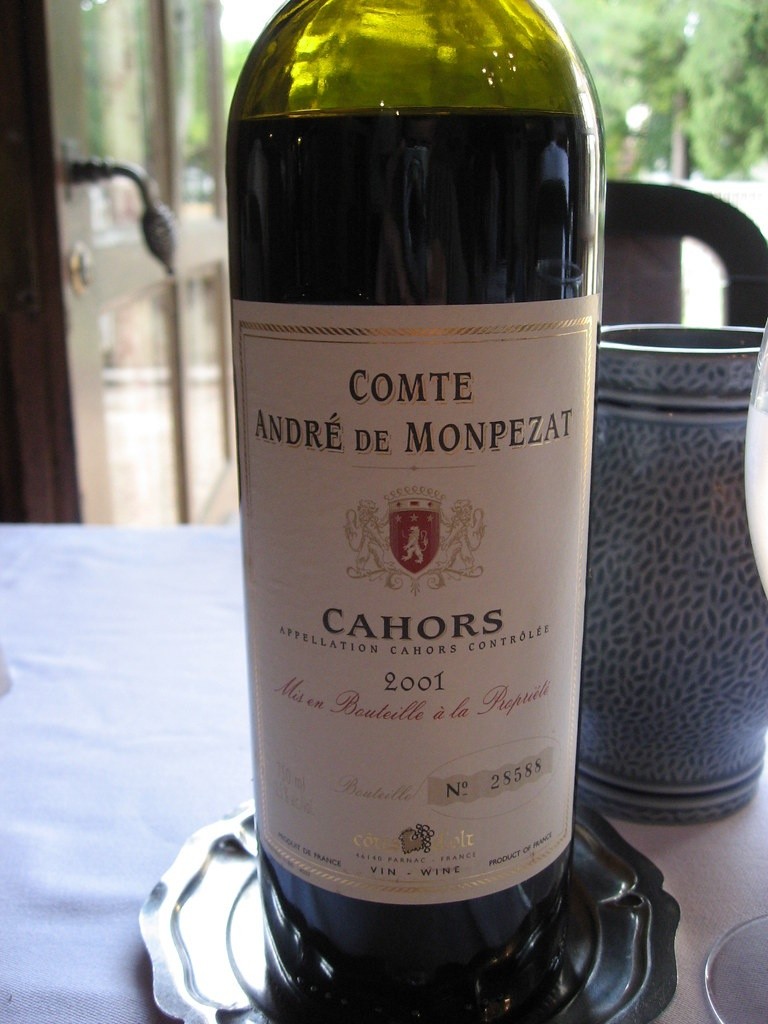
<box><xmin>0</xmin><ymin>522</ymin><xmax>768</xmax><ymax>1024</ymax></box>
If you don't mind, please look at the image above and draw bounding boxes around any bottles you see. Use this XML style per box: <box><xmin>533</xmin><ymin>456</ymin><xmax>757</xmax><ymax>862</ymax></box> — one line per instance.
<box><xmin>223</xmin><ymin>0</ymin><xmax>608</xmax><ymax>1024</ymax></box>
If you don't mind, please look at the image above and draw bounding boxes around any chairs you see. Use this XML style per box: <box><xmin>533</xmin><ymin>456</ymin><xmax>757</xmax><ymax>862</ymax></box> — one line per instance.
<box><xmin>606</xmin><ymin>174</ymin><xmax>768</xmax><ymax>326</ymax></box>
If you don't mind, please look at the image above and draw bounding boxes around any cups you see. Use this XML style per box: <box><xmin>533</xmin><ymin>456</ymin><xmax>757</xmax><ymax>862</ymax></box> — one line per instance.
<box><xmin>574</xmin><ymin>324</ymin><xmax>768</xmax><ymax>825</ymax></box>
